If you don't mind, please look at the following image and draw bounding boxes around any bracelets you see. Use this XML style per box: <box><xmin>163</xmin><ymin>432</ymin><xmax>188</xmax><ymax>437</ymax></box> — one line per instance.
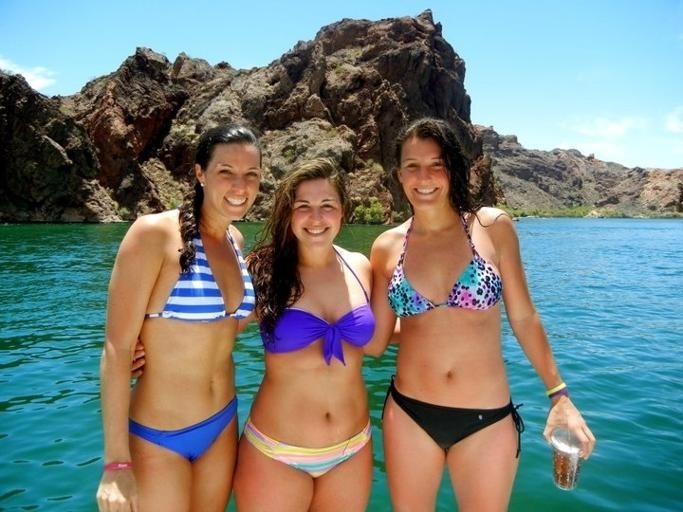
<box><xmin>546</xmin><ymin>382</ymin><xmax>568</xmax><ymax>399</ymax></box>
<box><xmin>102</xmin><ymin>461</ymin><xmax>133</xmax><ymax>472</ymax></box>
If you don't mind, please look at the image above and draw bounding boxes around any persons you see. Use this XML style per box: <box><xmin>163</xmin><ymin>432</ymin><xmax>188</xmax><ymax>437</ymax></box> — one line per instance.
<box><xmin>351</xmin><ymin>113</ymin><xmax>600</xmax><ymax>511</ymax></box>
<box><xmin>126</xmin><ymin>156</ymin><xmax>404</xmax><ymax>512</ymax></box>
<box><xmin>92</xmin><ymin>122</ymin><xmax>259</xmax><ymax>511</ymax></box>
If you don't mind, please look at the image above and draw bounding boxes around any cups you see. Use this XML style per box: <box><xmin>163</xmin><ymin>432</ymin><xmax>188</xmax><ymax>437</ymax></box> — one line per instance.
<box><xmin>550</xmin><ymin>428</ymin><xmax>583</xmax><ymax>491</ymax></box>
